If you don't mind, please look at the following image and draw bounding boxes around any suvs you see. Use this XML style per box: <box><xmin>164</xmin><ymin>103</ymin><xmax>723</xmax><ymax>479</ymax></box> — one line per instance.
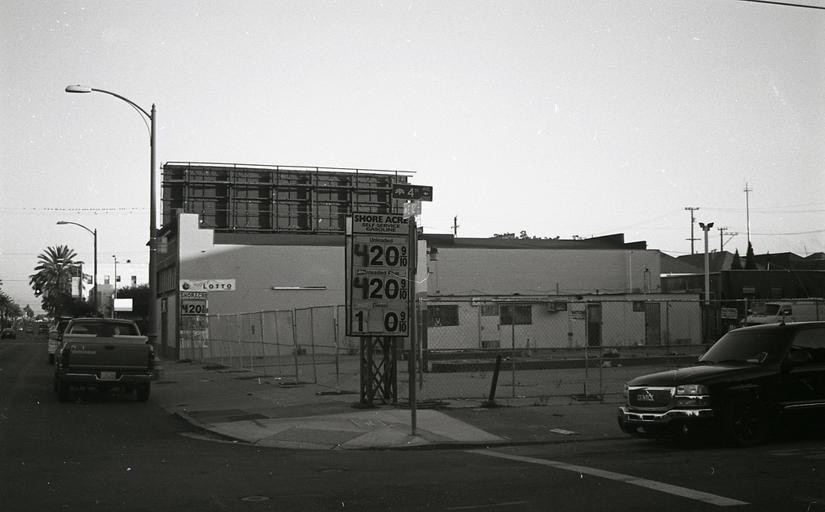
<box><xmin>617</xmin><ymin>317</ymin><xmax>825</xmax><ymax>448</ymax></box>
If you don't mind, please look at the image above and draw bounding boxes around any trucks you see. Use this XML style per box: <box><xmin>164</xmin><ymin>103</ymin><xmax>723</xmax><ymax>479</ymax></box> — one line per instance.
<box><xmin>739</xmin><ymin>297</ymin><xmax>824</xmax><ymax>331</ymax></box>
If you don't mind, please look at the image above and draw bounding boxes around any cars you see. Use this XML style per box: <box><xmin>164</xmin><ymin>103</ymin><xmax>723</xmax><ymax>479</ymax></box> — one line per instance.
<box><xmin>0</xmin><ymin>317</ymin><xmax>73</xmax><ymax>366</ymax></box>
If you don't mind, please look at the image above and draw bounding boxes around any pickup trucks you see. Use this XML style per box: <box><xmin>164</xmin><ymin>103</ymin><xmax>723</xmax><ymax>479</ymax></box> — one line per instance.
<box><xmin>52</xmin><ymin>316</ymin><xmax>158</xmax><ymax>403</ymax></box>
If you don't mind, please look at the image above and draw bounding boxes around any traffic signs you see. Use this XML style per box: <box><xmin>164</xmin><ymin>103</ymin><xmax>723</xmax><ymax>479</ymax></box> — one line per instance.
<box><xmin>393</xmin><ymin>183</ymin><xmax>432</xmax><ymax>202</ymax></box>
<box><xmin>401</xmin><ymin>199</ymin><xmax>422</xmax><ymax>221</ymax></box>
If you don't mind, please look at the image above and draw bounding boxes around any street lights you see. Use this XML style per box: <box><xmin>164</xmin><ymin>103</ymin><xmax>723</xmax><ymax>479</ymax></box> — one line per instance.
<box><xmin>61</xmin><ymin>83</ymin><xmax>161</xmax><ymax>372</ymax></box>
<box><xmin>111</xmin><ymin>254</ymin><xmax>133</xmax><ymax>319</ymax></box>
<box><xmin>698</xmin><ymin>221</ymin><xmax>719</xmax><ymax>307</ymax></box>
<box><xmin>55</xmin><ymin>221</ymin><xmax>97</xmax><ymax>317</ymax></box>
